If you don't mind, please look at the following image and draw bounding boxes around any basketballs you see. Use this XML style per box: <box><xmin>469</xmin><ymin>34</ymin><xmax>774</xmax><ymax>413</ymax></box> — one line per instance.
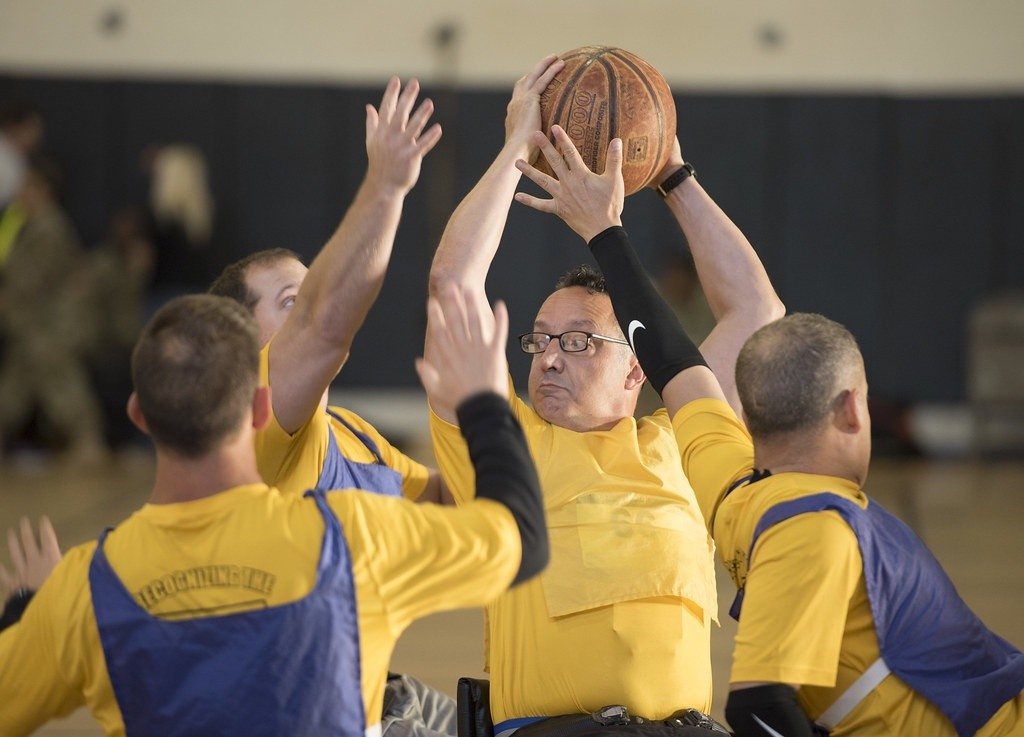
<box><xmin>533</xmin><ymin>45</ymin><xmax>678</xmax><ymax>196</ymax></box>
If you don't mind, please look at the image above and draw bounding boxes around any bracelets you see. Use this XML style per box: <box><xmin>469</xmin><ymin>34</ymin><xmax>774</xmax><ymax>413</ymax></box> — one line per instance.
<box><xmin>656</xmin><ymin>163</ymin><xmax>698</xmax><ymax>200</ymax></box>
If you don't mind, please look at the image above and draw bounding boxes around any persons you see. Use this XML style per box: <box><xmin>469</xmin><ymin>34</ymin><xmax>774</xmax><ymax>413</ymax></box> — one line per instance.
<box><xmin>0</xmin><ymin>277</ymin><xmax>551</xmax><ymax>737</ymax></box>
<box><xmin>203</xmin><ymin>75</ymin><xmax>457</xmax><ymax>737</ymax></box>
<box><xmin>514</xmin><ymin>126</ymin><xmax>1024</xmax><ymax>737</ymax></box>
<box><xmin>419</xmin><ymin>52</ymin><xmax>786</xmax><ymax>737</ymax></box>
<box><xmin>0</xmin><ymin>104</ymin><xmax>217</xmax><ymax>474</ymax></box>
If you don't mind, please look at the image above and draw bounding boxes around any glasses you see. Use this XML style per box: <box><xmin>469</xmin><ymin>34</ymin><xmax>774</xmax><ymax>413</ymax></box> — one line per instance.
<box><xmin>518</xmin><ymin>331</ymin><xmax>631</xmax><ymax>354</ymax></box>
